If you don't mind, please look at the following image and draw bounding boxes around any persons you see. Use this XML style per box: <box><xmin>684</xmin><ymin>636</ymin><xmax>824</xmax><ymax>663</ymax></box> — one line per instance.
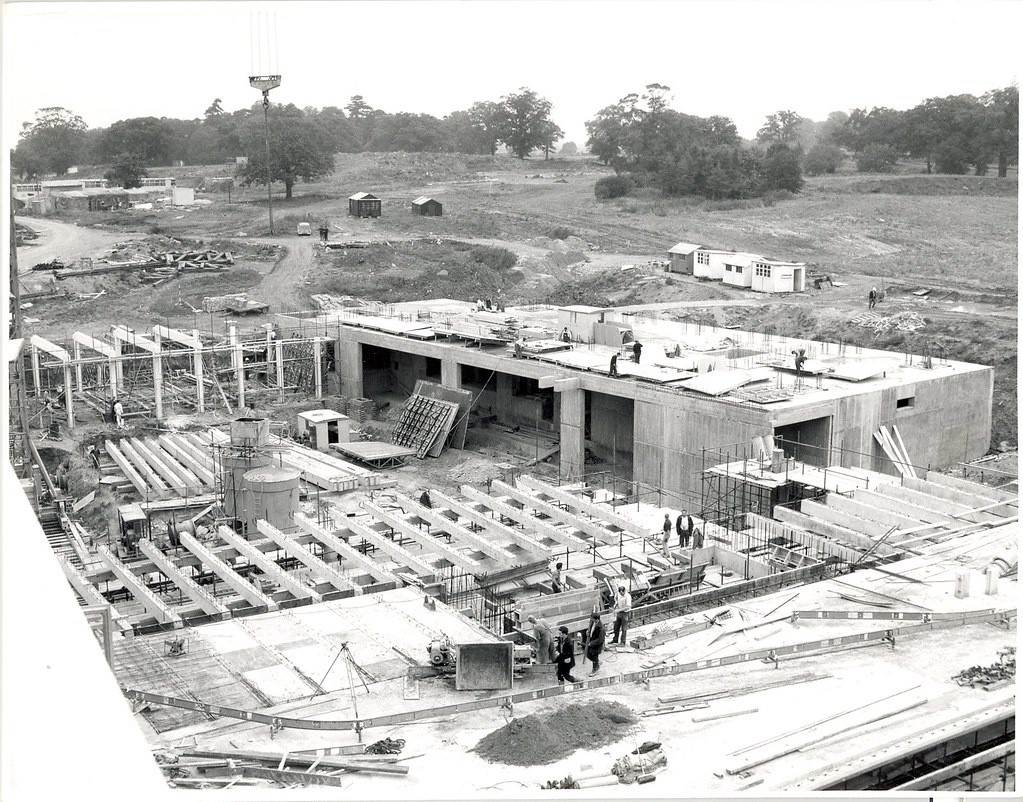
<box><xmin>792</xmin><ymin>348</ymin><xmax>807</xmax><ymax>373</ymax></box>
<box><xmin>319</xmin><ymin>225</ymin><xmax>328</xmax><ymax>241</ymax></box>
<box><xmin>90</xmin><ymin>446</ymin><xmax>101</xmax><ymax>469</ymax></box>
<box><xmin>515</xmin><ymin>337</ymin><xmax>526</xmax><ymax>360</ymax></box>
<box><xmin>551</xmin><ymin>563</ymin><xmax>562</xmax><ymax>593</ymax></box>
<box><xmin>57</xmin><ymin>385</ymin><xmax>63</xmax><ymax>406</ymax></box>
<box><xmin>661</xmin><ymin>514</ymin><xmax>672</xmax><ymax>558</ymax></box>
<box><xmin>555</xmin><ymin>626</ymin><xmax>583</xmax><ymax>688</ymax></box>
<box><xmin>676</xmin><ymin>510</ymin><xmax>693</xmax><ymax>547</ymax></box>
<box><xmin>528</xmin><ymin>615</ymin><xmax>556</xmax><ymax>664</ymax></box>
<box><xmin>586</xmin><ymin>613</ymin><xmax>606</xmax><ymax>677</ymax></box>
<box><xmin>477</xmin><ymin>297</ymin><xmax>492</xmax><ymax>311</ymax></box>
<box><xmin>633</xmin><ymin>340</ymin><xmax>643</xmax><ymax>364</ymax></box>
<box><xmin>114</xmin><ymin>401</ymin><xmax>123</xmax><ymax>426</ymax></box>
<box><xmin>607</xmin><ymin>353</ymin><xmax>621</xmax><ymax>378</ymax></box>
<box><xmin>562</xmin><ymin>327</ymin><xmax>572</xmax><ymax>350</ymax></box>
<box><xmin>608</xmin><ymin>586</ymin><xmax>632</xmax><ymax>647</ymax></box>
<box><xmin>50</xmin><ymin>421</ymin><xmax>59</xmax><ymax>439</ymax></box>
<box><xmin>88</xmin><ymin>199</ymin><xmax>122</xmax><ymax>211</ymax></box>
<box><xmin>44</xmin><ymin>392</ymin><xmax>50</xmax><ymax>406</ymax></box>
<box><xmin>869</xmin><ymin>287</ymin><xmax>877</xmax><ymax>309</ymax></box>
<box><xmin>692</xmin><ymin>528</ymin><xmax>703</xmax><ymax>549</ymax></box>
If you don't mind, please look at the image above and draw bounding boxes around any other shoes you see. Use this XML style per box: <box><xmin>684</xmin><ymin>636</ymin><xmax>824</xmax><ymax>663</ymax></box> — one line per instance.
<box><xmin>589</xmin><ymin>665</ymin><xmax>599</xmax><ymax>677</ymax></box>
<box><xmin>619</xmin><ymin>642</ymin><xmax>625</xmax><ymax>647</ymax></box>
<box><xmin>663</xmin><ymin>555</ymin><xmax>670</xmax><ymax>558</ymax></box>
<box><xmin>608</xmin><ymin>640</ymin><xmax>617</xmax><ymax>644</ymax></box>
<box><xmin>571</xmin><ymin>676</ymin><xmax>574</xmax><ymax>683</ymax></box>
